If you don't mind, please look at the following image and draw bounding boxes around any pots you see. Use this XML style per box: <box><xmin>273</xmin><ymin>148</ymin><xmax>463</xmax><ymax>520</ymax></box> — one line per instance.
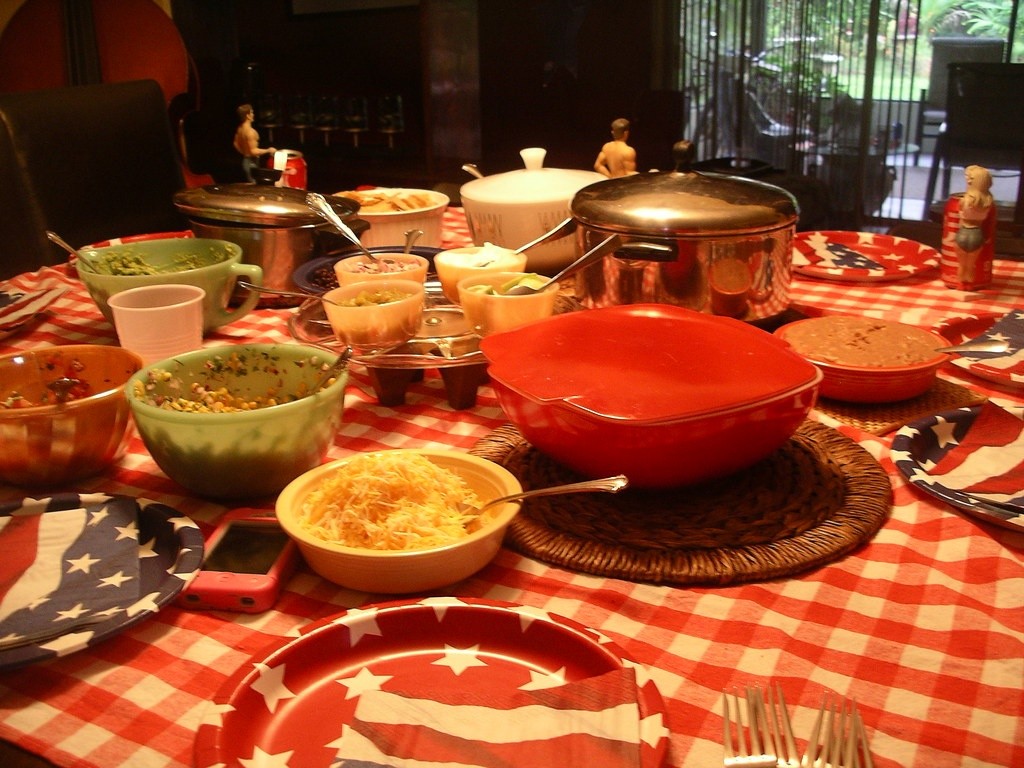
<box><xmin>478</xmin><ymin>304</ymin><xmax>824</xmax><ymax>490</ymax></box>
<box><xmin>171</xmin><ymin>166</ymin><xmax>370</xmax><ymax>309</ymax></box>
<box><xmin>459</xmin><ymin>148</ymin><xmax>610</xmax><ymax>279</ymax></box>
<box><xmin>567</xmin><ymin>140</ymin><xmax>800</xmax><ymax>326</ymax></box>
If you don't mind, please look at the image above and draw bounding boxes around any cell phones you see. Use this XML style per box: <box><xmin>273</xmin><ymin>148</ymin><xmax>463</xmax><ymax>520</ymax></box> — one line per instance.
<box><xmin>174</xmin><ymin>508</ymin><xmax>300</xmax><ymax>614</ymax></box>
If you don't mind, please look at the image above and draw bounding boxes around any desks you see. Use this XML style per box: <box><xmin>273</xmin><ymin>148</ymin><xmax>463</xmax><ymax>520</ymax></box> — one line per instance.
<box><xmin>790</xmin><ymin>139</ymin><xmax>918</xmax><ymax>197</ymax></box>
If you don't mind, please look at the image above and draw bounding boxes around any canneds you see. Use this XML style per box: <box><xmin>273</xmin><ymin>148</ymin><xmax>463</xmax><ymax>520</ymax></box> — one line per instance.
<box><xmin>267</xmin><ymin>149</ymin><xmax>307</xmax><ymax>190</ymax></box>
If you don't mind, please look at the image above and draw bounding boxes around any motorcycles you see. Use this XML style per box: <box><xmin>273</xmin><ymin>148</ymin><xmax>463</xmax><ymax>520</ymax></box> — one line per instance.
<box><xmin>682</xmin><ymin>30</ymin><xmax>895</xmax><ymax>216</ymax></box>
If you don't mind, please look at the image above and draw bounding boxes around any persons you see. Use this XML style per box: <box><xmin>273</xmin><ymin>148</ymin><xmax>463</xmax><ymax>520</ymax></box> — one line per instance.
<box><xmin>736</xmin><ymin>72</ymin><xmax>818</xmax><ymax>189</ymax></box>
<box><xmin>234</xmin><ymin>104</ymin><xmax>277</xmax><ymax>184</ymax></box>
<box><xmin>955</xmin><ymin>164</ymin><xmax>993</xmax><ymax>291</ymax></box>
<box><xmin>596</xmin><ymin>118</ymin><xmax>637</xmax><ymax>182</ymax></box>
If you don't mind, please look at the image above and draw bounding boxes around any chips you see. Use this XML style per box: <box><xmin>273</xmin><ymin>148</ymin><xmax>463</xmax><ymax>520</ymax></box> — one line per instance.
<box><xmin>338</xmin><ymin>189</ymin><xmax>436</xmax><ymax>214</ymax></box>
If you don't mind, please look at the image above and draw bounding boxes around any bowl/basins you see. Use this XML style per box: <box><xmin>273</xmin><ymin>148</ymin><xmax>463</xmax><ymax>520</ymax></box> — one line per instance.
<box><xmin>432</xmin><ymin>244</ymin><xmax>528</xmax><ymax>306</ymax></box>
<box><xmin>457</xmin><ymin>272</ymin><xmax>561</xmax><ymax>339</ymax></box>
<box><xmin>0</xmin><ymin>343</ymin><xmax>145</xmax><ymax>492</ymax></box>
<box><xmin>332</xmin><ymin>187</ymin><xmax>451</xmax><ymax>250</ymax></box>
<box><xmin>274</xmin><ymin>447</ymin><xmax>524</xmax><ymax>595</ymax></box>
<box><xmin>124</xmin><ymin>343</ymin><xmax>349</xmax><ymax>504</ymax></box>
<box><xmin>322</xmin><ymin>279</ymin><xmax>425</xmax><ymax>356</ymax></box>
<box><xmin>770</xmin><ymin>315</ymin><xmax>954</xmax><ymax>403</ymax></box>
<box><xmin>333</xmin><ymin>252</ymin><xmax>429</xmax><ymax>288</ymax></box>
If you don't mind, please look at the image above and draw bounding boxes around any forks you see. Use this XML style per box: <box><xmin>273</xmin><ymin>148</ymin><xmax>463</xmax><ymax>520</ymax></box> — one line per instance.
<box><xmin>720</xmin><ymin>682</ymin><xmax>873</xmax><ymax>768</ymax></box>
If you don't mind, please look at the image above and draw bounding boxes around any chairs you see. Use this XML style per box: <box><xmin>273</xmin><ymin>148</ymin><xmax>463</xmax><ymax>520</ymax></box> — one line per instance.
<box><xmin>922</xmin><ymin>63</ymin><xmax>1024</xmax><ymax>222</ymax></box>
<box><xmin>712</xmin><ymin>66</ymin><xmax>798</xmax><ymax>170</ymax></box>
<box><xmin>916</xmin><ymin>37</ymin><xmax>1006</xmax><ymax>165</ymax></box>
<box><xmin>0</xmin><ymin>79</ymin><xmax>185</xmax><ymax>281</ymax></box>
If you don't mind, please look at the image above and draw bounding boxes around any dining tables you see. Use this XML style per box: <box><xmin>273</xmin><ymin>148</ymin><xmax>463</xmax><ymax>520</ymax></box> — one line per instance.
<box><xmin>0</xmin><ymin>205</ymin><xmax>1024</xmax><ymax>768</ymax></box>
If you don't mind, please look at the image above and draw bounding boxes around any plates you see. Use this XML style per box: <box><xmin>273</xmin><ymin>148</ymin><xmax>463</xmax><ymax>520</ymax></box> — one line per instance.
<box><xmin>0</xmin><ymin>491</ymin><xmax>205</xmax><ymax>671</ymax></box>
<box><xmin>791</xmin><ymin>229</ymin><xmax>942</xmax><ymax>284</ymax></box>
<box><xmin>888</xmin><ymin>403</ymin><xmax>1024</xmax><ymax>533</ymax></box>
<box><xmin>191</xmin><ymin>595</ymin><xmax>671</xmax><ymax>768</ymax></box>
<box><xmin>930</xmin><ymin>312</ymin><xmax>1024</xmax><ymax>391</ymax></box>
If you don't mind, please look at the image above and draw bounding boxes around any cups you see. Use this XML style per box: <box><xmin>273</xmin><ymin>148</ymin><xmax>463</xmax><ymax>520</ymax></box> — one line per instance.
<box><xmin>76</xmin><ymin>237</ymin><xmax>263</xmax><ymax>332</ymax></box>
<box><xmin>106</xmin><ymin>283</ymin><xmax>207</xmax><ymax>370</ymax></box>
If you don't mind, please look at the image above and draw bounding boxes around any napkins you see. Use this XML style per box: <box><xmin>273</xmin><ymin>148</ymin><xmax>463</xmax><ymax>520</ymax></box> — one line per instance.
<box><xmin>958</xmin><ymin>309</ymin><xmax>1024</xmax><ymax>372</ymax></box>
<box><xmin>792</xmin><ymin>238</ymin><xmax>889</xmax><ymax>269</ymax></box>
<box><xmin>329</xmin><ymin>669</ymin><xmax>640</xmax><ymax>768</ymax></box>
<box><xmin>0</xmin><ymin>287</ymin><xmax>70</xmax><ymax>330</ymax></box>
<box><xmin>0</xmin><ymin>497</ymin><xmax>140</xmax><ymax>652</ymax></box>
<box><xmin>925</xmin><ymin>401</ymin><xmax>1024</xmax><ymax>508</ymax></box>
